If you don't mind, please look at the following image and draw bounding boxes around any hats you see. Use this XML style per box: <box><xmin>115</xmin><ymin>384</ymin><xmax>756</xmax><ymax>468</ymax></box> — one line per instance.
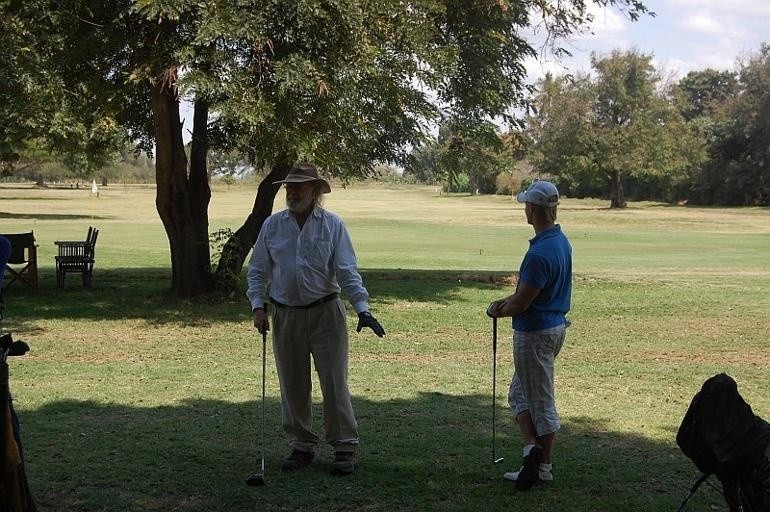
<box><xmin>273</xmin><ymin>163</ymin><xmax>331</xmax><ymax>194</ymax></box>
<box><xmin>517</xmin><ymin>181</ymin><xmax>560</xmax><ymax>209</ymax></box>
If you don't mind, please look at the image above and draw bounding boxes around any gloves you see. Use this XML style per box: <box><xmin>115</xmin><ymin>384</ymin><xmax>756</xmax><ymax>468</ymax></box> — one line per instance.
<box><xmin>355</xmin><ymin>312</ymin><xmax>385</xmax><ymax>338</ymax></box>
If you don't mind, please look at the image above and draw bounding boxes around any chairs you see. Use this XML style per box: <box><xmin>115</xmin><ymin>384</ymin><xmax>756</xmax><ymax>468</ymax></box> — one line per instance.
<box><xmin>0</xmin><ymin>229</ymin><xmax>39</xmax><ymax>289</ymax></box>
<box><xmin>55</xmin><ymin>226</ymin><xmax>99</xmax><ymax>290</ymax></box>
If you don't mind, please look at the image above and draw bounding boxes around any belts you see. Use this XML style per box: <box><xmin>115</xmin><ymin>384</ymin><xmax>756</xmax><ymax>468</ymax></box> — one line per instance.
<box><xmin>270</xmin><ymin>292</ymin><xmax>338</xmax><ymax>310</ymax></box>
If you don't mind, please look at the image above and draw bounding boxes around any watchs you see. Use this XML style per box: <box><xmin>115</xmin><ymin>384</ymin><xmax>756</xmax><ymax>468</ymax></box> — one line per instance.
<box><xmin>360</xmin><ymin>312</ymin><xmax>372</xmax><ymax>318</ymax></box>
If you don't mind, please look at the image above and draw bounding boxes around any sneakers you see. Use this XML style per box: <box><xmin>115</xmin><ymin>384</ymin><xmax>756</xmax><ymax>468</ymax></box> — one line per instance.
<box><xmin>504</xmin><ymin>443</ymin><xmax>553</xmax><ymax>490</ymax></box>
<box><xmin>283</xmin><ymin>449</ymin><xmax>315</xmax><ymax>466</ymax></box>
<box><xmin>329</xmin><ymin>451</ymin><xmax>355</xmax><ymax>472</ymax></box>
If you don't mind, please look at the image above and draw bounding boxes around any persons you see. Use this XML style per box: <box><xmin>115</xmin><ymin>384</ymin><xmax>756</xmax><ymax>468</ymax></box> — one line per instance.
<box><xmin>244</xmin><ymin>160</ymin><xmax>386</xmax><ymax>478</ymax></box>
<box><xmin>487</xmin><ymin>179</ymin><xmax>574</xmax><ymax>489</ymax></box>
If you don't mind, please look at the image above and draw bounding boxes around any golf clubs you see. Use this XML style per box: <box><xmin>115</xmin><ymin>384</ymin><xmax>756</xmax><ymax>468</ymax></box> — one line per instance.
<box><xmin>246</xmin><ymin>302</ymin><xmax>268</xmax><ymax>486</ymax></box>
<box><xmin>491</xmin><ymin>317</ymin><xmax>504</xmax><ymax>466</ymax></box>
